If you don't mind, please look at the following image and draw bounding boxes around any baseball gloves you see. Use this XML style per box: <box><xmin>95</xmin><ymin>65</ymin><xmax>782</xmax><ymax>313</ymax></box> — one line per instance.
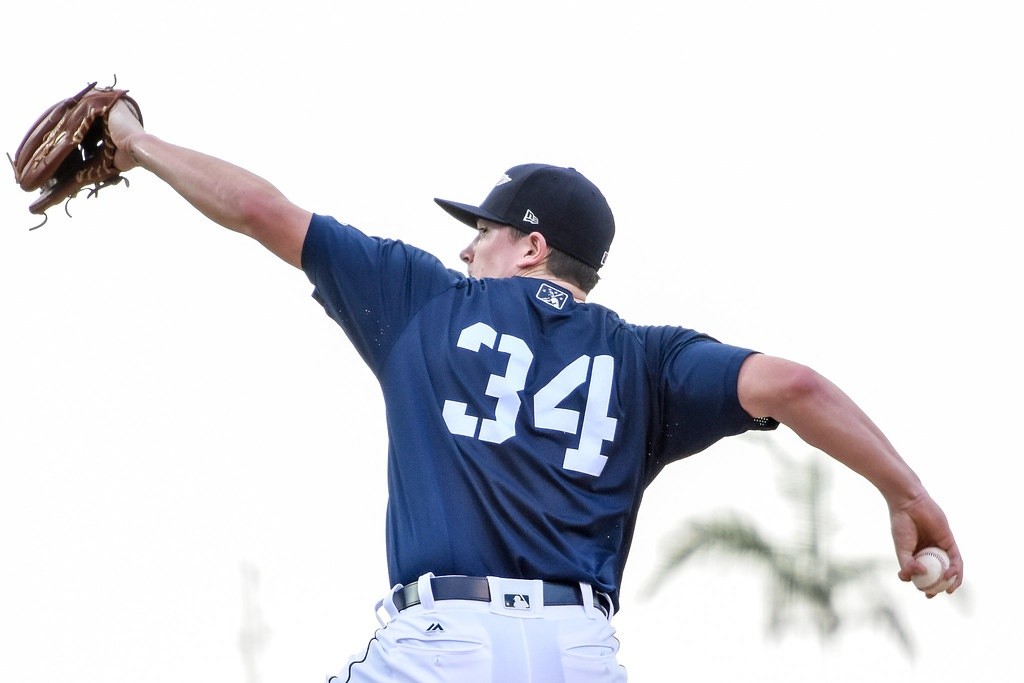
<box><xmin>5</xmin><ymin>71</ymin><xmax>143</xmax><ymax>231</ymax></box>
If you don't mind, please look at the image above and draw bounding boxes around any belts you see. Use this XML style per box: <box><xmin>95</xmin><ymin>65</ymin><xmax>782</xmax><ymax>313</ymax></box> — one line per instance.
<box><xmin>393</xmin><ymin>577</ymin><xmax>608</xmax><ymax>618</ymax></box>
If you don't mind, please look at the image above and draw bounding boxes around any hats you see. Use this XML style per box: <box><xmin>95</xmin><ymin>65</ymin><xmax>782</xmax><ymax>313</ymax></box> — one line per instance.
<box><xmin>435</xmin><ymin>163</ymin><xmax>615</xmax><ymax>272</ymax></box>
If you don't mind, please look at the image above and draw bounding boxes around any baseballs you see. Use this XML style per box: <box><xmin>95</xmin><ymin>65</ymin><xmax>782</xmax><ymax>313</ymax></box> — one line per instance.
<box><xmin>910</xmin><ymin>546</ymin><xmax>958</xmax><ymax>594</ymax></box>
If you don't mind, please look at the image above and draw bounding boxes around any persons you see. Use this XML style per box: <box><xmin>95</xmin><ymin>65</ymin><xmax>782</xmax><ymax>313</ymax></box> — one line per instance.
<box><xmin>6</xmin><ymin>73</ymin><xmax>962</xmax><ymax>683</ymax></box>
